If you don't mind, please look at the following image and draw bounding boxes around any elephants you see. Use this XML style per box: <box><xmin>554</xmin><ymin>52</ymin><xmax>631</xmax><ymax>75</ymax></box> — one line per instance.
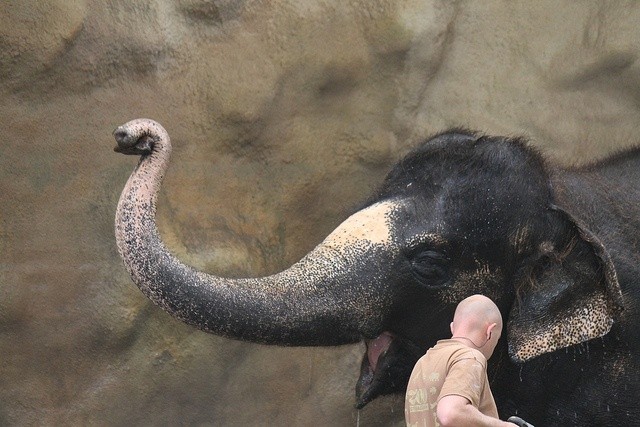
<box><xmin>110</xmin><ymin>118</ymin><xmax>639</xmax><ymax>427</ymax></box>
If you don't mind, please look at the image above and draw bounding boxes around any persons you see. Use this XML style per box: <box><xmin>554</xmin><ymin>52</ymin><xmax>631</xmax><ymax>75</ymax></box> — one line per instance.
<box><xmin>403</xmin><ymin>294</ymin><xmax>521</xmax><ymax>427</ymax></box>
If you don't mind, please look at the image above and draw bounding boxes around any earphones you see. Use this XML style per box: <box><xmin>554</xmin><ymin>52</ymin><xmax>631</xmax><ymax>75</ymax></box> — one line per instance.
<box><xmin>488</xmin><ymin>332</ymin><xmax>492</xmax><ymax>340</ymax></box>
